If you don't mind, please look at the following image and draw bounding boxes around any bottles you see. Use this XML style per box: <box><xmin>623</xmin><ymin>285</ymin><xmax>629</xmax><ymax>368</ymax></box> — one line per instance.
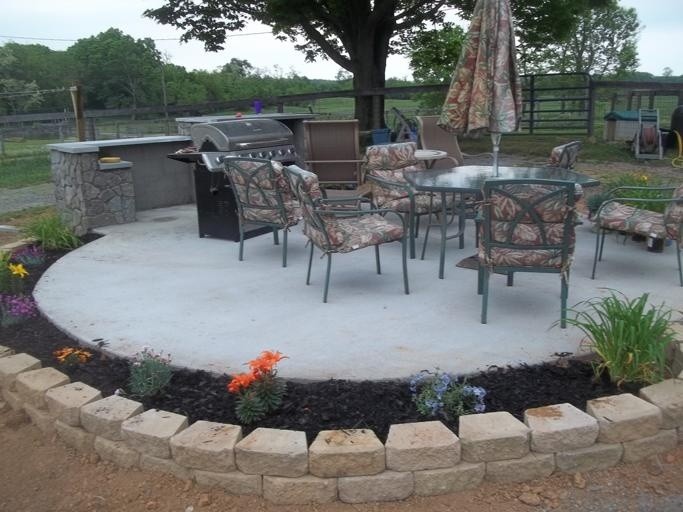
<box><xmin>253</xmin><ymin>98</ymin><xmax>261</xmax><ymax>113</ymax></box>
<box><xmin>647</xmin><ymin>233</ymin><xmax>665</xmax><ymax>252</ymax></box>
<box><xmin>276</xmin><ymin>102</ymin><xmax>283</xmax><ymax>113</ymax></box>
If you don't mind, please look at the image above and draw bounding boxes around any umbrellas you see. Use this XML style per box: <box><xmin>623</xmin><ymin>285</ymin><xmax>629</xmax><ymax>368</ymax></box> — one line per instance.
<box><xmin>435</xmin><ymin>0</ymin><xmax>523</xmax><ymax>175</ymax></box>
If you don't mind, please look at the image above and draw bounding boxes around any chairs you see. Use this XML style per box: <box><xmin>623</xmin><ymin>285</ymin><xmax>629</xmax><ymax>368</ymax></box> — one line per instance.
<box><xmin>414</xmin><ymin>111</ymin><xmax>494</xmax><ymax>230</ymax></box>
<box><xmin>474</xmin><ymin>179</ymin><xmax>580</xmax><ymax>330</ymax></box>
<box><xmin>283</xmin><ymin>162</ymin><xmax>413</xmax><ymax>305</ymax></box>
<box><xmin>542</xmin><ymin>141</ymin><xmax>580</xmax><ymax>166</ymax></box>
<box><xmin>216</xmin><ymin>156</ymin><xmax>326</xmax><ymax>268</ymax></box>
<box><xmin>305</xmin><ymin>116</ymin><xmax>370</xmax><ymax>199</ymax></box>
<box><xmin>590</xmin><ymin>175</ymin><xmax>682</xmax><ymax>291</ymax></box>
<box><xmin>362</xmin><ymin>141</ymin><xmax>470</xmax><ymax>279</ymax></box>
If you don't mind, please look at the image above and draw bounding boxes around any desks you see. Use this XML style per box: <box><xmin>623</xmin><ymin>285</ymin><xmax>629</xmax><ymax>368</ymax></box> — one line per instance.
<box><xmin>412</xmin><ymin>149</ymin><xmax>447</xmax><ymax>240</ymax></box>
<box><xmin>398</xmin><ymin>164</ymin><xmax>601</xmax><ymax>292</ymax></box>
